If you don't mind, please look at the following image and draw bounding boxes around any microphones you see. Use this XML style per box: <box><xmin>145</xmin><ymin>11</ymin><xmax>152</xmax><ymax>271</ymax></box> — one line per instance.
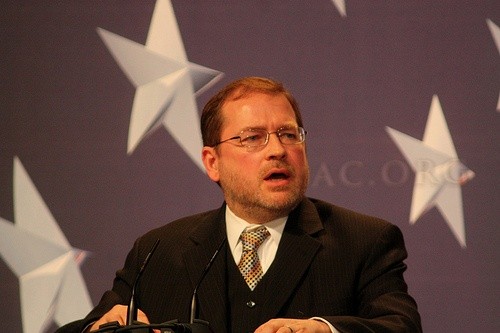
<box><xmin>171</xmin><ymin>235</ymin><xmax>227</xmax><ymax>333</ymax></box>
<box><xmin>117</xmin><ymin>238</ymin><xmax>160</xmax><ymax>333</ymax></box>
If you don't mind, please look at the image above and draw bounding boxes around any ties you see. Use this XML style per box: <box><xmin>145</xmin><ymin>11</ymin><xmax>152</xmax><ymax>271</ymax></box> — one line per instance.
<box><xmin>238</xmin><ymin>226</ymin><xmax>270</xmax><ymax>289</ymax></box>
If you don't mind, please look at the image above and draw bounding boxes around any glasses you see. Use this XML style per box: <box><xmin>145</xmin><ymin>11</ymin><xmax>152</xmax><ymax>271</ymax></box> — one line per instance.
<box><xmin>210</xmin><ymin>124</ymin><xmax>308</xmax><ymax>147</ymax></box>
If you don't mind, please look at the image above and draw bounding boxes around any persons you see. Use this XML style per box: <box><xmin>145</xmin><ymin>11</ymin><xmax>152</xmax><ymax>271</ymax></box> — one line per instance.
<box><xmin>83</xmin><ymin>77</ymin><xmax>423</xmax><ymax>333</ymax></box>
